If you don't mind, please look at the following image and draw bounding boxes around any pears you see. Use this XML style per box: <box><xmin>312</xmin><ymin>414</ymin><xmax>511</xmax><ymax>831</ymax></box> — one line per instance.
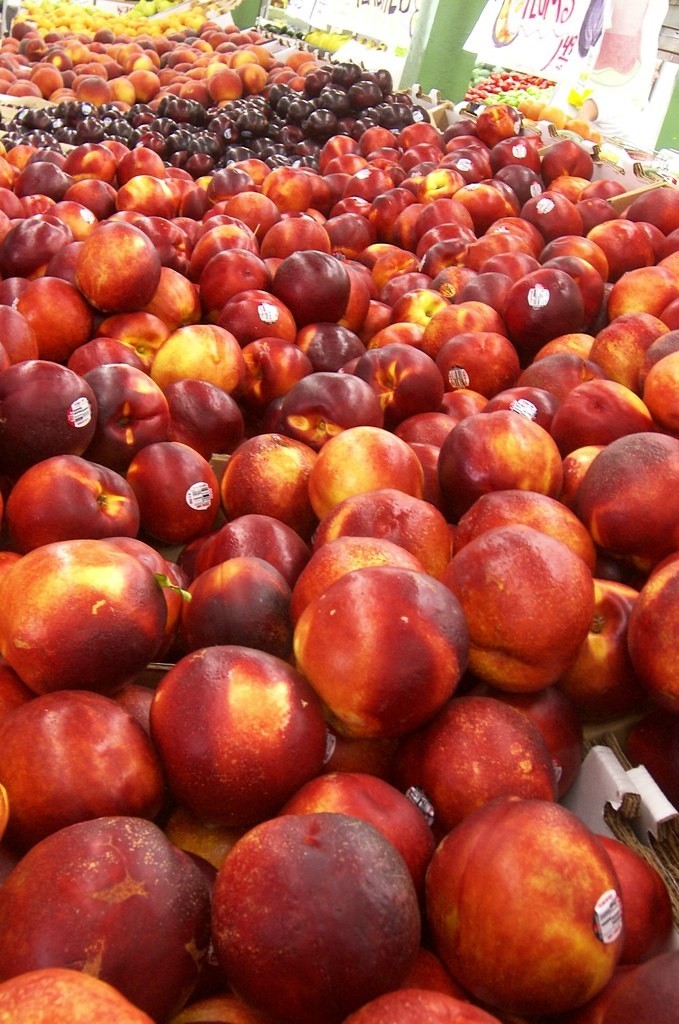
<box><xmin>21</xmin><ymin>0</ymin><xmax>178</xmax><ymax>16</ymax></box>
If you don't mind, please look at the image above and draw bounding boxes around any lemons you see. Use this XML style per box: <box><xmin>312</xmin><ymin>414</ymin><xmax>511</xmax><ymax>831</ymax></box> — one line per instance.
<box><xmin>10</xmin><ymin>4</ymin><xmax>218</xmax><ymax>38</ymax></box>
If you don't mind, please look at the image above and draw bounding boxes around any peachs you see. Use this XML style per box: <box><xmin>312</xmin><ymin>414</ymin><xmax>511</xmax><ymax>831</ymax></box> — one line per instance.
<box><xmin>0</xmin><ymin>20</ymin><xmax>679</xmax><ymax>1024</ymax></box>
